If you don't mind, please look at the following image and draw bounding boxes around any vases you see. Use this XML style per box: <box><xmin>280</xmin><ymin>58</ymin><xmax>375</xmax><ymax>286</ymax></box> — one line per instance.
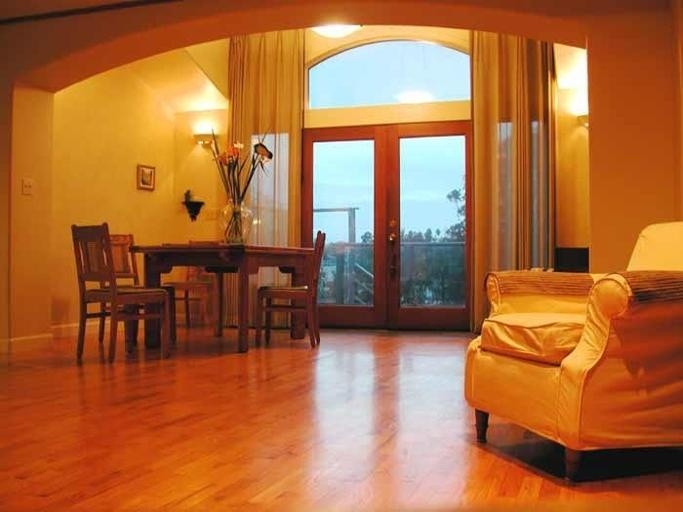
<box><xmin>208</xmin><ymin>199</ymin><xmax>257</xmax><ymax>248</ymax></box>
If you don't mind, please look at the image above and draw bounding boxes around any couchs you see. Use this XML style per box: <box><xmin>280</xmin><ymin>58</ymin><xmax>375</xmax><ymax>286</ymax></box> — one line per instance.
<box><xmin>465</xmin><ymin>222</ymin><xmax>682</xmax><ymax>482</ymax></box>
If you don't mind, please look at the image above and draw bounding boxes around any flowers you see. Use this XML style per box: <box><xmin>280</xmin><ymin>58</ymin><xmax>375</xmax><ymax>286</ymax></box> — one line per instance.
<box><xmin>202</xmin><ymin>126</ymin><xmax>273</xmax><ymax>196</ymax></box>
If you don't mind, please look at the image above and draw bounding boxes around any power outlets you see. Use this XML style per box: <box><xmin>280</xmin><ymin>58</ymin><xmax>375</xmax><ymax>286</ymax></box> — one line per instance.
<box><xmin>22</xmin><ymin>176</ymin><xmax>36</xmax><ymax>197</ymax></box>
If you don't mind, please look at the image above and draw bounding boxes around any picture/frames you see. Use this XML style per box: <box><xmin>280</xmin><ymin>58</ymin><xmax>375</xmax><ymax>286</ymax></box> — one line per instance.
<box><xmin>137</xmin><ymin>165</ymin><xmax>158</xmax><ymax>190</ymax></box>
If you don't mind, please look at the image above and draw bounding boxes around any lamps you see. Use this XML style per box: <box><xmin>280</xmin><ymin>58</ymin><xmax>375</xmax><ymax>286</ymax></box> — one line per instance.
<box><xmin>193</xmin><ymin>119</ymin><xmax>217</xmax><ymax>147</ymax></box>
<box><xmin>570</xmin><ymin>91</ymin><xmax>588</xmax><ymax>128</ymax></box>
<box><xmin>182</xmin><ymin>190</ymin><xmax>204</xmax><ymax>221</ymax></box>
<box><xmin>311</xmin><ymin>25</ymin><xmax>358</xmax><ymax>38</ymax></box>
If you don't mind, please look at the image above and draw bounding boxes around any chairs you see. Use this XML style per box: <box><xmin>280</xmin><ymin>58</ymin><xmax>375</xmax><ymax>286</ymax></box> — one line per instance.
<box><xmin>101</xmin><ymin>234</ymin><xmax>171</xmax><ymax>352</ymax></box>
<box><xmin>256</xmin><ymin>230</ymin><xmax>329</xmax><ymax>348</ymax></box>
<box><xmin>314</xmin><ymin>230</ymin><xmax>322</xmax><ymax>247</ymax></box>
<box><xmin>70</xmin><ymin>222</ymin><xmax>173</xmax><ymax>362</ymax></box>
<box><xmin>165</xmin><ymin>240</ymin><xmax>222</xmax><ymax>335</ymax></box>
